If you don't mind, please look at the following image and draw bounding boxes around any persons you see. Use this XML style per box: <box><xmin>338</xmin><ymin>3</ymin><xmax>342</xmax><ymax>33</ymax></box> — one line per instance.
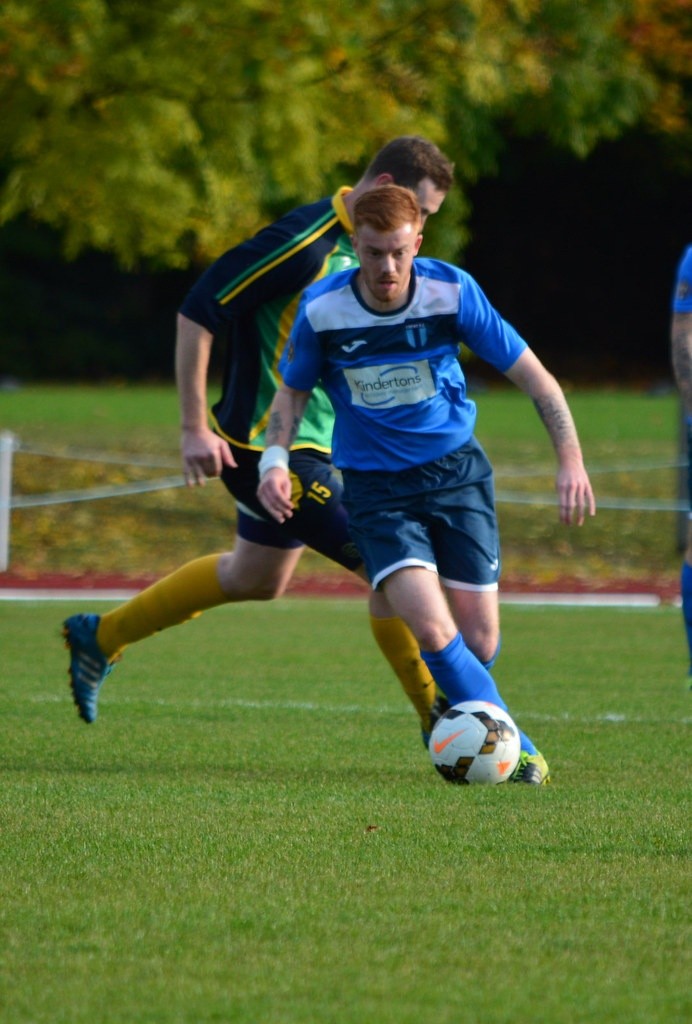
<box><xmin>63</xmin><ymin>137</ymin><xmax>461</xmax><ymax>750</ymax></box>
<box><xmin>671</xmin><ymin>244</ymin><xmax>692</xmax><ymax>690</ymax></box>
<box><xmin>257</xmin><ymin>187</ymin><xmax>596</xmax><ymax>786</ymax></box>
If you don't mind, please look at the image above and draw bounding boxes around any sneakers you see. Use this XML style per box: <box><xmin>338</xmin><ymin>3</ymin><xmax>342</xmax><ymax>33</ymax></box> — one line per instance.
<box><xmin>420</xmin><ymin>695</ymin><xmax>450</xmax><ymax>747</ymax></box>
<box><xmin>60</xmin><ymin>613</ymin><xmax>115</xmax><ymax>724</ymax></box>
<box><xmin>507</xmin><ymin>736</ymin><xmax>551</xmax><ymax>787</ymax></box>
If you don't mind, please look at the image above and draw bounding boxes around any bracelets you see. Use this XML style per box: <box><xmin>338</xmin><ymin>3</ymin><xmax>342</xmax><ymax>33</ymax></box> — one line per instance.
<box><xmin>259</xmin><ymin>445</ymin><xmax>289</xmax><ymax>480</ymax></box>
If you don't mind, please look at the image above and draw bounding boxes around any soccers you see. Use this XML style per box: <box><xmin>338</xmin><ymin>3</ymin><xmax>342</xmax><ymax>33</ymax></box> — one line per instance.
<box><xmin>429</xmin><ymin>700</ymin><xmax>521</xmax><ymax>785</ymax></box>
